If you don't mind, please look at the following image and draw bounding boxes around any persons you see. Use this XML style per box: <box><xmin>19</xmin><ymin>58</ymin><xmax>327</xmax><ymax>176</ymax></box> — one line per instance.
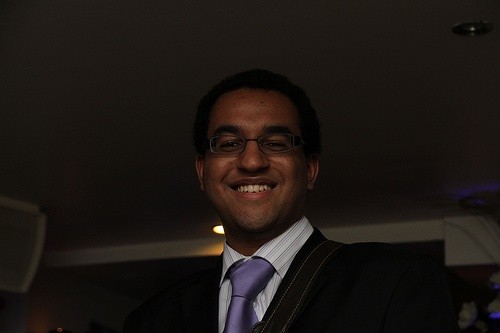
<box><xmin>120</xmin><ymin>67</ymin><xmax>459</xmax><ymax>333</ymax></box>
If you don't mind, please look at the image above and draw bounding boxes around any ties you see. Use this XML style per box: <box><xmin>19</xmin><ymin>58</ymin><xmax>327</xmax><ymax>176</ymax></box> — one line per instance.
<box><xmin>222</xmin><ymin>256</ymin><xmax>276</xmax><ymax>333</ymax></box>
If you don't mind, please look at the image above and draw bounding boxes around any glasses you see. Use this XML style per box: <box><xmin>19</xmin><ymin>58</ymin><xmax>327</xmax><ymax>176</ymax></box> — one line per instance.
<box><xmin>200</xmin><ymin>132</ymin><xmax>306</xmax><ymax>156</ymax></box>
